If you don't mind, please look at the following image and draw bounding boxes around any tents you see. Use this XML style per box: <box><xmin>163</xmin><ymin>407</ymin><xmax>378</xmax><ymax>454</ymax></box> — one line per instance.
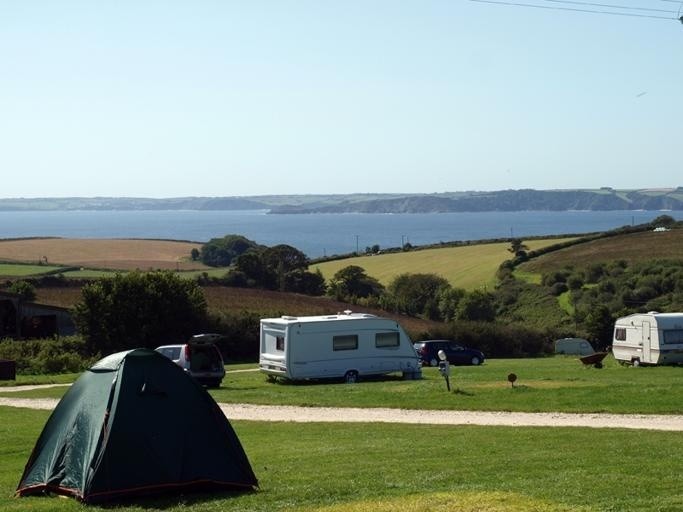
<box><xmin>14</xmin><ymin>348</ymin><xmax>262</xmax><ymax>504</ymax></box>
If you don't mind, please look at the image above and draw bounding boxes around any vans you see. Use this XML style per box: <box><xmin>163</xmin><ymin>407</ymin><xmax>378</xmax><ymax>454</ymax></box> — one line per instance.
<box><xmin>154</xmin><ymin>334</ymin><xmax>225</xmax><ymax>387</ymax></box>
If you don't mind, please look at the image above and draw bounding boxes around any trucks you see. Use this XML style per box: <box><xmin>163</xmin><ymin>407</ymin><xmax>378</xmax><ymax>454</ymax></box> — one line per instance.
<box><xmin>612</xmin><ymin>311</ymin><xmax>683</xmax><ymax>366</ymax></box>
<box><xmin>259</xmin><ymin>309</ymin><xmax>422</xmax><ymax>384</ymax></box>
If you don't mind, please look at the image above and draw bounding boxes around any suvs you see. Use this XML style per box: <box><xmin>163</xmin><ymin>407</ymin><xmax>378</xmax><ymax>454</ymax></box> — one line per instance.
<box><xmin>413</xmin><ymin>339</ymin><xmax>485</xmax><ymax>367</ymax></box>
